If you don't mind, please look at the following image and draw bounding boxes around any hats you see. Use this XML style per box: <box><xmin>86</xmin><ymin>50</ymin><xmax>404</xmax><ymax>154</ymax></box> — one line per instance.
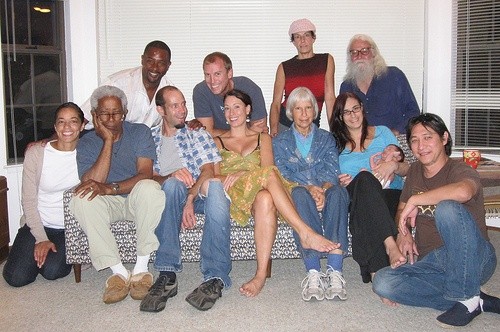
<box><xmin>289</xmin><ymin>19</ymin><xmax>315</xmax><ymax>37</ymax></box>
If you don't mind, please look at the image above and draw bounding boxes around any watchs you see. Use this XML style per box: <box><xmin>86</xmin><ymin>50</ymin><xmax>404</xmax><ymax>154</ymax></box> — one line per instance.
<box><xmin>111</xmin><ymin>182</ymin><xmax>122</xmax><ymax>195</ymax></box>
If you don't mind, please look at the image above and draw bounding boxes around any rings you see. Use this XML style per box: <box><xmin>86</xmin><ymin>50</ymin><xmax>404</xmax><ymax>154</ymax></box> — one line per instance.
<box><xmin>90</xmin><ymin>187</ymin><xmax>93</xmax><ymax>190</ymax></box>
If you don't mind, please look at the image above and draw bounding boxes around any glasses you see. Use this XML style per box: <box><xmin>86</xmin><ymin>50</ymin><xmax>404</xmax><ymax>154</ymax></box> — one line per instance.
<box><xmin>97</xmin><ymin>112</ymin><xmax>125</xmax><ymax>123</ymax></box>
<box><xmin>340</xmin><ymin>103</ymin><xmax>362</xmax><ymax>115</ymax></box>
<box><xmin>350</xmin><ymin>48</ymin><xmax>371</xmax><ymax>57</ymax></box>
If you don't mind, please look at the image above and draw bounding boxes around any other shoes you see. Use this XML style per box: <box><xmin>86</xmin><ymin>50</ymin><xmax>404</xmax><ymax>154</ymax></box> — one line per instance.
<box><xmin>103</xmin><ymin>273</ymin><xmax>131</xmax><ymax>304</ymax></box>
<box><xmin>436</xmin><ymin>302</ymin><xmax>482</xmax><ymax>326</ymax></box>
<box><xmin>479</xmin><ymin>291</ymin><xmax>500</xmax><ymax>313</ymax></box>
<box><xmin>130</xmin><ymin>271</ymin><xmax>153</xmax><ymax>300</ymax></box>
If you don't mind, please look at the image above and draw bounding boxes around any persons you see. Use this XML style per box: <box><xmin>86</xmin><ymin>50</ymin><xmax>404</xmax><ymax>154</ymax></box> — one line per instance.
<box><xmin>270</xmin><ymin>86</ymin><xmax>350</xmax><ymax>303</ymax></box>
<box><xmin>362</xmin><ymin>145</ymin><xmax>404</xmax><ymax>192</ymax></box>
<box><xmin>137</xmin><ymin>84</ymin><xmax>233</xmax><ymax>312</ymax></box>
<box><xmin>191</xmin><ymin>52</ymin><xmax>269</xmax><ymax>145</ymax></box>
<box><xmin>24</xmin><ymin>40</ymin><xmax>206</xmax><ymax>151</ymax></box>
<box><xmin>370</xmin><ymin>112</ymin><xmax>497</xmax><ymax>326</ymax></box>
<box><xmin>336</xmin><ymin>42</ymin><xmax>419</xmax><ymax>139</ymax></box>
<box><xmin>328</xmin><ymin>92</ymin><xmax>409</xmax><ymax>308</ymax></box>
<box><xmin>270</xmin><ymin>30</ymin><xmax>337</xmax><ymax>139</ymax></box>
<box><xmin>202</xmin><ymin>91</ymin><xmax>341</xmax><ymax>297</ymax></box>
<box><xmin>3</xmin><ymin>102</ymin><xmax>84</xmax><ymax>287</ymax></box>
<box><xmin>74</xmin><ymin>87</ymin><xmax>166</xmax><ymax>302</ymax></box>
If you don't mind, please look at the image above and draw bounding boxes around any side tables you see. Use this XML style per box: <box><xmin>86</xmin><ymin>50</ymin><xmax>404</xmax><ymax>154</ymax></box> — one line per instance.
<box><xmin>0</xmin><ymin>176</ymin><xmax>10</xmax><ymax>264</ymax></box>
<box><xmin>452</xmin><ymin>156</ymin><xmax>500</xmax><ymax>231</ymax></box>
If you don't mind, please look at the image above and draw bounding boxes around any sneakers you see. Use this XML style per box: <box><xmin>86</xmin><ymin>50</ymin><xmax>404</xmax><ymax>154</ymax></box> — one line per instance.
<box><xmin>185</xmin><ymin>278</ymin><xmax>224</xmax><ymax>311</ymax></box>
<box><xmin>140</xmin><ymin>275</ymin><xmax>178</xmax><ymax>312</ymax></box>
<box><xmin>301</xmin><ymin>269</ymin><xmax>327</xmax><ymax>301</ymax></box>
<box><xmin>324</xmin><ymin>264</ymin><xmax>348</xmax><ymax>300</ymax></box>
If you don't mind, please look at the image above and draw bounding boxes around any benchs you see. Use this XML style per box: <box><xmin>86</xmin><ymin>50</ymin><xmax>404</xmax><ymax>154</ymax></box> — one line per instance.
<box><xmin>63</xmin><ymin>134</ymin><xmax>418</xmax><ymax>282</ymax></box>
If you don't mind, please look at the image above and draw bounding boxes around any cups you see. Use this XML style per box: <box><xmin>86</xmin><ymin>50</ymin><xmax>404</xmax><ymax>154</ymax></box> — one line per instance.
<box><xmin>464</xmin><ymin>150</ymin><xmax>480</xmax><ymax>170</ymax></box>
